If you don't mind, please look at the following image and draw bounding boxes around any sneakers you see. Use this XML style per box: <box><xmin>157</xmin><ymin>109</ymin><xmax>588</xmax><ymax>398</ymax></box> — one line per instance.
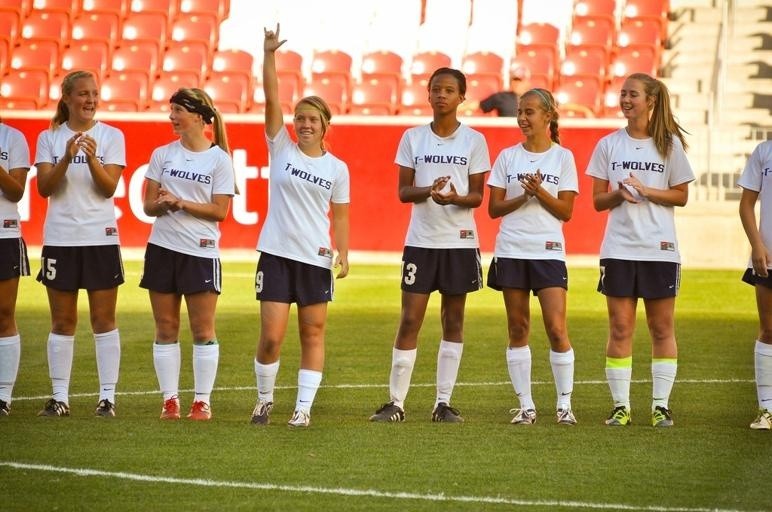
<box><xmin>160</xmin><ymin>394</ymin><xmax>180</xmax><ymax>418</ymax></box>
<box><xmin>95</xmin><ymin>399</ymin><xmax>116</xmax><ymax>419</ymax></box>
<box><xmin>0</xmin><ymin>399</ymin><xmax>9</xmax><ymax>416</ymax></box>
<box><xmin>750</xmin><ymin>408</ymin><xmax>771</xmax><ymax>430</ymax></box>
<box><xmin>369</xmin><ymin>401</ymin><xmax>404</xmax><ymax>422</ymax></box>
<box><xmin>431</xmin><ymin>401</ymin><xmax>463</xmax><ymax>422</ymax></box>
<box><xmin>555</xmin><ymin>407</ymin><xmax>577</xmax><ymax>425</ymax></box>
<box><xmin>605</xmin><ymin>406</ymin><xmax>631</xmax><ymax>426</ymax></box>
<box><xmin>187</xmin><ymin>401</ymin><xmax>212</xmax><ymax>420</ymax></box>
<box><xmin>37</xmin><ymin>399</ymin><xmax>70</xmax><ymax>418</ymax></box>
<box><xmin>651</xmin><ymin>406</ymin><xmax>674</xmax><ymax>427</ymax></box>
<box><xmin>509</xmin><ymin>408</ymin><xmax>537</xmax><ymax>424</ymax></box>
<box><xmin>251</xmin><ymin>398</ymin><xmax>273</xmax><ymax>425</ymax></box>
<box><xmin>287</xmin><ymin>408</ymin><xmax>310</xmax><ymax>427</ymax></box>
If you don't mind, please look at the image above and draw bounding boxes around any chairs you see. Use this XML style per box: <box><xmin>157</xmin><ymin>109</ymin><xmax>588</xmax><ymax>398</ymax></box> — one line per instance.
<box><xmin>2</xmin><ymin>0</ymin><xmax>668</xmax><ymax>119</ymax></box>
<box><xmin>665</xmin><ymin>1</ymin><xmax>770</xmax><ymax>202</ymax></box>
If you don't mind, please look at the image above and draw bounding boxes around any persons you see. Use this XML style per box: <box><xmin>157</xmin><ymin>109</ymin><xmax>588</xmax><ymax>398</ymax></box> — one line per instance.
<box><xmin>0</xmin><ymin>119</ymin><xmax>31</xmax><ymax>414</ymax></box>
<box><xmin>736</xmin><ymin>140</ymin><xmax>771</xmax><ymax>429</ymax></box>
<box><xmin>368</xmin><ymin>67</ymin><xmax>491</xmax><ymax>426</ymax></box>
<box><xmin>584</xmin><ymin>72</ymin><xmax>696</xmax><ymax>428</ymax></box>
<box><xmin>34</xmin><ymin>71</ymin><xmax>128</xmax><ymax>418</ymax></box>
<box><xmin>140</xmin><ymin>87</ymin><xmax>239</xmax><ymax>421</ymax></box>
<box><xmin>486</xmin><ymin>88</ymin><xmax>579</xmax><ymax>427</ymax></box>
<box><xmin>252</xmin><ymin>20</ymin><xmax>351</xmax><ymax>428</ymax></box>
<box><xmin>479</xmin><ymin>63</ymin><xmax>532</xmax><ymax>116</ymax></box>
